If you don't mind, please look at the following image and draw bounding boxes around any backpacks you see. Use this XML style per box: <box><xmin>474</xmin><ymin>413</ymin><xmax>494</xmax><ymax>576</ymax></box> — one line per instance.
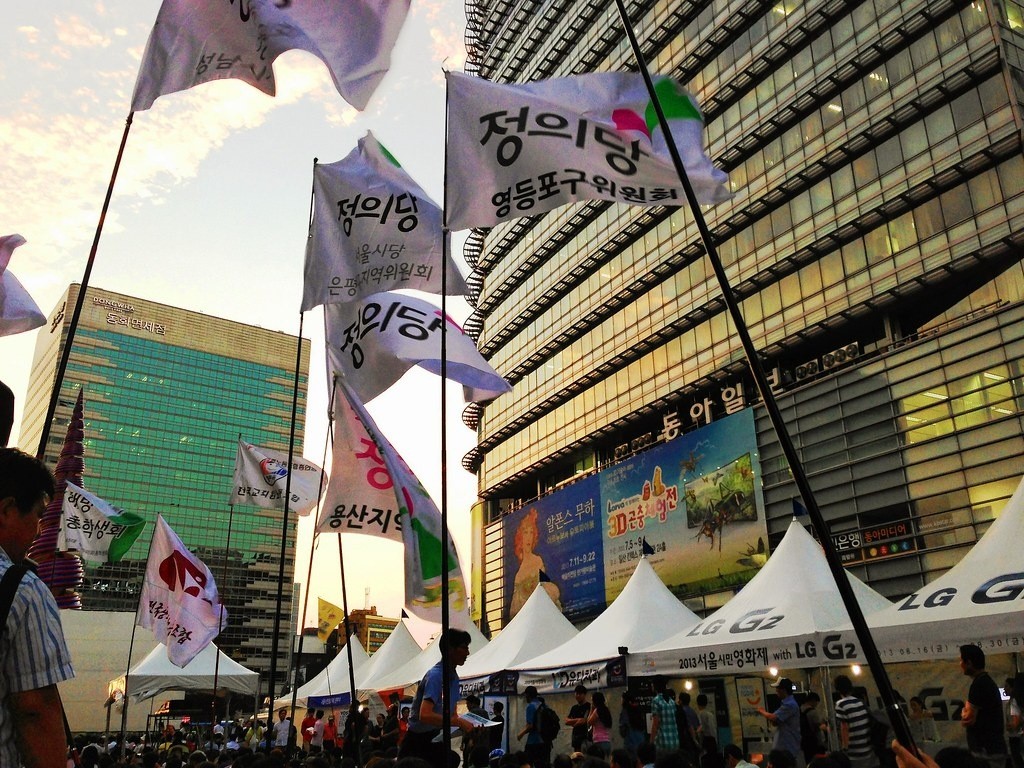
<box><xmin>533</xmin><ymin>704</ymin><xmax>560</xmax><ymax>742</ymax></box>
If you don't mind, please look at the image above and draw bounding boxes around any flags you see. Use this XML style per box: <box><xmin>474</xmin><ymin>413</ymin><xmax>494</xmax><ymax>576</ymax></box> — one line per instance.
<box><xmin>444</xmin><ymin>71</ymin><xmax>735</xmax><ymax>233</ymax></box>
<box><xmin>322</xmin><ymin>292</ymin><xmax>512</xmax><ymax>406</ymax></box>
<box><xmin>131</xmin><ymin>0</ymin><xmax>412</xmax><ymax>111</ymax></box>
<box><xmin>227</xmin><ymin>438</ymin><xmax>328</xmax><ymax>516</ymax></box>
<box><xmin>316</xmin><ymin>377</ymin><xmax>468</xmax><ymax>608</ymax></box>
<box><xmin>56</xmin><ymin>479</ymin><xmax>145</xmax><ymax>562</ymax></box>
<box><xmin>301</xmin><ymin>130</ymin><xmax>471</xmax><ymax>312</ymax></box>
<box><xmin>136</xmin><ymin>512</ymin><xmax>229</xmax><ymax>668</ymax></box>
<box><xmin>0</xmin><ymin>234</ymin><xmax>47</xmax><ymax>338</ymax></box>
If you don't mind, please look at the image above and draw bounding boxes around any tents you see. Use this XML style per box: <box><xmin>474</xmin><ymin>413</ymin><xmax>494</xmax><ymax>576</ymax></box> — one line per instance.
<box><xmin>273</xmin><ymin>472</ymin><xmax>1024</xmax><ymax>711</ymax></box>
<box><xmin>106</xmin><ymin>639</ymin><xmax>261</xmax><ymax>756</ymax></box>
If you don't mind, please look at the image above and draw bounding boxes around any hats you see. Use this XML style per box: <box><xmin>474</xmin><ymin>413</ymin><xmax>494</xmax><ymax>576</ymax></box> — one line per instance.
<box><xmin>522</xmin><ymin>687</ymin><xmax>537</xmax><ymax>696</ymax></box>
<box><xmin>771</xmin><ymin>677</ymin><xmax>793</xmax><ymax>692</ymax></box>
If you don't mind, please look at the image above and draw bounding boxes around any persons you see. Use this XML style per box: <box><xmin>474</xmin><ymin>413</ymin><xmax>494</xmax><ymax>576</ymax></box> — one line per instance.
<box><xmin>71</xmin><ymin>628</ymin><xmax>1024</xmax><ymax>768</ymax></box>
<box><xmin>0</xmin><ymin>448</ymin><xmax>75</xmax><ymax>768</ymax></box>
